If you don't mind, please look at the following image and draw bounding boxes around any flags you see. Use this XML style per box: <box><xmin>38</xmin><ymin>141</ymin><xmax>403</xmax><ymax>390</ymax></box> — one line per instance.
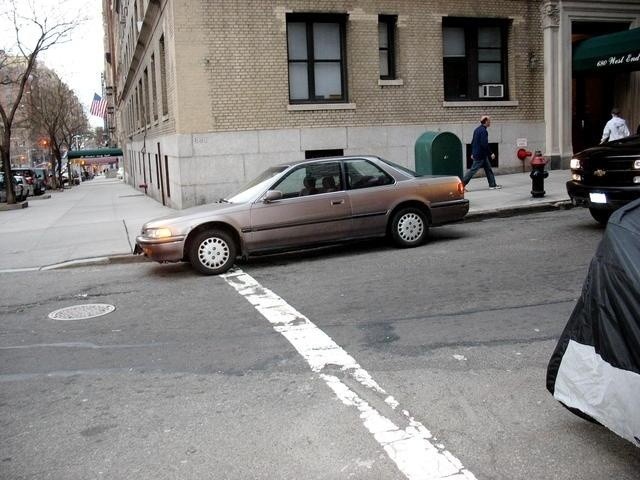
<box><xmin>89</xmin><ymin>92</ymin><xmax>107</xmax><ymax>118</ymax></box>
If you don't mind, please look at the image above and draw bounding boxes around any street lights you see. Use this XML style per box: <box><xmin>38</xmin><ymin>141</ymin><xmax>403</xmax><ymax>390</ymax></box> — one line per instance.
<box><xmin>10</xmin><ymin>154</ymin><xmax>25</xmax><ymax>166</ymax></box>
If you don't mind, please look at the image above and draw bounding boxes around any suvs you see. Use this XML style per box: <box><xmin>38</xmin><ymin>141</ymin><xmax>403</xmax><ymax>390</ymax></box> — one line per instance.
<box><xmin>566</xmin><ymin>124</ymin><xmax>640</xmax><ymax>224</ymax></box>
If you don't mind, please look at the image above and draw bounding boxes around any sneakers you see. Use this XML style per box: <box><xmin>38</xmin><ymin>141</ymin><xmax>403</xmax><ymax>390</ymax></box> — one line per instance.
<box><xmin>488</xmin><ymin>184</ymin><xmax>502</xmax><ymax>190</ymax></box>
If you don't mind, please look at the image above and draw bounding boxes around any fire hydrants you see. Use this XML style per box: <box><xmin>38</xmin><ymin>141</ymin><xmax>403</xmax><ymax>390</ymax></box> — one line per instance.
<box><xmin>530</xmin><ymin>150</ymin><xmax>550</xmax><ymax>198</ymax></box>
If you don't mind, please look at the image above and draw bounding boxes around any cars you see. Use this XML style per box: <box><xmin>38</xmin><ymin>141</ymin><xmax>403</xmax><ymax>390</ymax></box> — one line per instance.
<box><xmin>0</xmin><ymin>162</ymin><xmax>86</xmax><ymax>203</ymax></box>
<box><xmin>135</xmin><ymin>155</ymin><xmax>471</xmax><ymax>276</ymax></box>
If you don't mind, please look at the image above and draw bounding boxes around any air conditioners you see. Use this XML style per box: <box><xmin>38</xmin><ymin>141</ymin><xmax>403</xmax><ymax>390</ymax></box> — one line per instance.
<box><xmin>478</xmin><ymin>83</ymin><xmax>504</xmax><ymax>98</ymax></box>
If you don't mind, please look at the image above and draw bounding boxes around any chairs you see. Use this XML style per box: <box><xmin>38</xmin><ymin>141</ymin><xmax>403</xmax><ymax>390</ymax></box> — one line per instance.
<box><xmin>299</xmin><ymin>175</ymin><xmax>318</xmax><ymax>196</ymax></box>
<box><xmin>322</xmin><ymin>176</ymin><xmax>338</xmax><ymax>193</ymax></box>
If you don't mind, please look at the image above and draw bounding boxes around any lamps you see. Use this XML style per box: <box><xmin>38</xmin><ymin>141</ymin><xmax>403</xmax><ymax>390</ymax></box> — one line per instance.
<box><xmin>528</xmin><ymin>51</ymin><xmax>537</xmax><ymax>72</ymax></box>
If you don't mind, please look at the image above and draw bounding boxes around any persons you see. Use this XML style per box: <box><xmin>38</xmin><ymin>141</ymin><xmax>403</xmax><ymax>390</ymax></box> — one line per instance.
<box><xmin>596</xmin><ymin>108</ymin><xmax>631</xmax><ymax>144</ymax></box>
<box><xmin>458</xmin><ymin>114</ymin><xmax>501</xmax><ymax>191</ymax></box>
